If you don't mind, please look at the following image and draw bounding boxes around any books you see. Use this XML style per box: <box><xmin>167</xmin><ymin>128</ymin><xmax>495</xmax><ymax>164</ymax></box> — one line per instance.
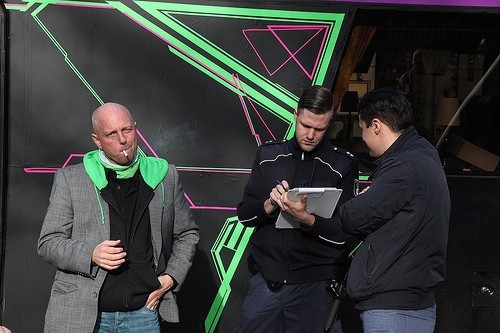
<box><xmin>274</xmin><ymin>186</ymin><xmax>343</xmax><ymax>228</ymax></box>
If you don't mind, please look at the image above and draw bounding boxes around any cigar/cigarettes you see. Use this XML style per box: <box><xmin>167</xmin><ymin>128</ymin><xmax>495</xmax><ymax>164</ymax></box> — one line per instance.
<box><xmin>122</xmin><ymin>150</ymin><xmax>129</xmax><ymax>158</ymax></box>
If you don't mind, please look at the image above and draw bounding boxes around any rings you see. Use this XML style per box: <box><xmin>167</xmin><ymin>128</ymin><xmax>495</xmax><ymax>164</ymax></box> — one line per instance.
<box><xmin>156</xmin><ymin>300</ymin><xmax>160</xmax><ymax>305</ymax></box>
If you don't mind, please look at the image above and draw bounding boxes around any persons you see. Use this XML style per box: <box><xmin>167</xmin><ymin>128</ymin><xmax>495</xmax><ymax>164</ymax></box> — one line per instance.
<box><xmin>336</xmin><ymin>86</ymin><xmax>451</xmax><ymax>333</ymax></box>
<box><xmin>232</xmin><ymin>84</ymin><xmax>361</xmax><ymax>333</ymax></box>
<box><xmin>36</xmin><ymin>101</ymin><xmax>201</xmax><ymax>333</ymax></box>
<box><xmin>397</xmin><ymin>48</ymin><xmax>441</xmax><ymax>144</ymax></box>
<box><xmin>459</xmin><ymin>32</ymin><xmax>500</xmax><ymax>175</ymax></box>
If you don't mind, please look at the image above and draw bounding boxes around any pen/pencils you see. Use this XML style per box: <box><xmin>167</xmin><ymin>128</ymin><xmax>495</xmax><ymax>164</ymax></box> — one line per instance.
<box><xmin>275</xmin><ymin>179</ymin><xmax>287</xmax><ymax>192</ymax></box>
